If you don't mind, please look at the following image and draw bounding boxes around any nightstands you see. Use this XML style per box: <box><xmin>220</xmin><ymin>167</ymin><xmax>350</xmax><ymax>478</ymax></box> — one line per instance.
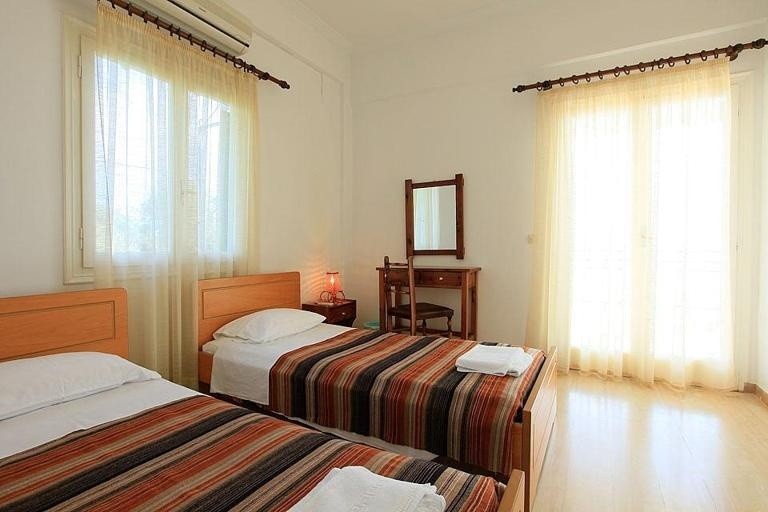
<box><xmin>301</xmin><ymin>299</ymin><xmax>356</xmax><ymax>329</ymax></box>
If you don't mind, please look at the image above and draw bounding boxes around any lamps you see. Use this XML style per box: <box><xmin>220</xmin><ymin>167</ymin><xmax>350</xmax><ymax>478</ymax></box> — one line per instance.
<box><xmin>319</xmin><ymin>272</ymin><xmax>346</xmax><ymax>305</ymax></box>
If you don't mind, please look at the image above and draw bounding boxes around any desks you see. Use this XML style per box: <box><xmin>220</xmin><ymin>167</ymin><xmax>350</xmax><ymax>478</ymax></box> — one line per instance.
<box><xmin>375</xmin><ymin>266</ymin><xmax>481</xmax><ymax>341</ymax></box>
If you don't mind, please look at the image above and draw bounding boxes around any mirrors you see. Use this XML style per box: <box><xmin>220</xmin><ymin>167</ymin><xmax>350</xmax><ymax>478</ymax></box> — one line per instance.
<box><xmin>404</xmin><ymin>173</ymin><xmax>465</xmax><ymax>261</ymax></box>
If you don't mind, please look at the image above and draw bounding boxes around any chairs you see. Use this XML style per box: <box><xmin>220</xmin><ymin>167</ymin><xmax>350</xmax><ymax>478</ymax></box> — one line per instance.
<box><xmin>383</xmin><ymin>255</ymin><xmax>454</xmax><ymax>339</ymax></box>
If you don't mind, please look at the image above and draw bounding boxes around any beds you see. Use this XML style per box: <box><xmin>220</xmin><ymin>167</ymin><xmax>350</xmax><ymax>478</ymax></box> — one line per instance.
<box><xmin>0</xmin><ymin>287</ymin><xmax>527</xmax><ymax>512</ymax></box>
<box><xmin>197</xmin><ymin>271</ymin><xmax>559</xmax><ymax>512</ymax></box>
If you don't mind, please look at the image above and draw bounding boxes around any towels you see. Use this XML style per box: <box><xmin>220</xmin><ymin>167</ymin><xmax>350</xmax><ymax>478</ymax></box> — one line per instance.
<box><xmin>454</xmin><ymin>344</ymin><xmax>533</xmax><ymax>379</ymax></box>
<box><xmin>287</xmin><ymin>465</ymin><xmax>446</xmax><ymax>512</ymax></box>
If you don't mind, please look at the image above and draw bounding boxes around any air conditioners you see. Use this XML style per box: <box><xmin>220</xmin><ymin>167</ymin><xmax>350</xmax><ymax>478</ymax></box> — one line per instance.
<box><xmin>126</xmin><ymin>0</ymin><xmax>254</xmax><ymax>58</ymax></box>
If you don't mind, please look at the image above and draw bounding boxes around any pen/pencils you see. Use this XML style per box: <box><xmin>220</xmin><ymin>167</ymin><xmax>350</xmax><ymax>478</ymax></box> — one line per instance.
<box><xmin>314</xmin><ymin>303</ymin><xmax>334</xmax><ymax>306</ymax></box>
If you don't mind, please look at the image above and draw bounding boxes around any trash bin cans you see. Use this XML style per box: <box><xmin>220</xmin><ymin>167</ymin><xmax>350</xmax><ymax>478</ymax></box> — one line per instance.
<box><xmin>363</xmin><ymin>320</ymin><xmax>380</xmax><ymax>329</ymax></box>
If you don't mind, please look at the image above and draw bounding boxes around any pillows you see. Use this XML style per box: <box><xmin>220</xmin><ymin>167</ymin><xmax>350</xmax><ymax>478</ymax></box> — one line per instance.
<box><xmin>211</xmin><ymin>306</ymin><xmax>327</xmax><ymax>343</ymax></box>
<box><xmin>0</xmin><ymin>350</ymin><xmax>163</xmax><ymax>422</ymax></box>
